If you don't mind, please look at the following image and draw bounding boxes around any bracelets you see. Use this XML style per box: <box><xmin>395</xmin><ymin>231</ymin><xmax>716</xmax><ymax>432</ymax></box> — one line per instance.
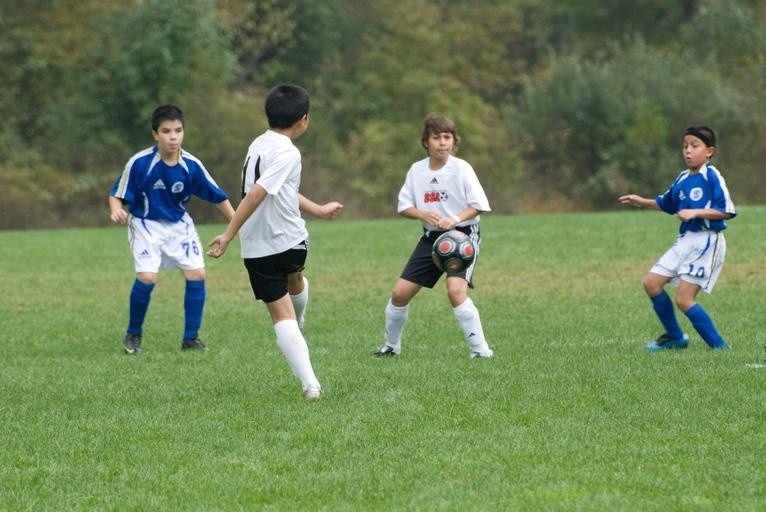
<box><xmin>452</xmin><ymin>215</ymin><xmax>461</xmax><ymax>225</ymax></box>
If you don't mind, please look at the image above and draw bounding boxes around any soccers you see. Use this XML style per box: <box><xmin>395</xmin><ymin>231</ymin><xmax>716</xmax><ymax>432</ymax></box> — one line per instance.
<box><xmin>432</xmin><ymin>231</ymin><xmax>475</xmax><ymax>274</ymax></box>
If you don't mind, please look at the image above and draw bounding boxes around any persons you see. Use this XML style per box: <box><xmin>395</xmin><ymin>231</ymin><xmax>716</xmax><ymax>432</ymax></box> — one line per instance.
<box><xmin>373</xmin><ymin>114</ymin><xmax>495</xmax><ymax>360</ymax></box>
<box><xmin>617</xmin><ymin>125</ymin><xmax>738</xmax><ymax>352</ymax></box>
<box><xmin>107</xmin><ymin>104</ymin><xmax>236</xmax><ymax>360</ymax></box>
<box><xmin>204</xmin><ymin>83</ymin><xmax>346</xmax><ymax>401</ymax></box>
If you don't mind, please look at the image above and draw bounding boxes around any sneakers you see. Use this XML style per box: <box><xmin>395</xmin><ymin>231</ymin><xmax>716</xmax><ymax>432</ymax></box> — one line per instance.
<box><xmin>182</xmin><ymin>338</ymin><xmax>206</xmax><ymax>351</ymax></box>
<box><xmin>302</xmin><ymin>388</ymin><xmax>322</xmax><ymax>400</ymax></box>
<box><xmin>471</xmin><ymin>350</ymin><xmax>492</xmax><ymax>357</ymax></box>
<box><xmin>123</xmin><ymin>334</ymin><xmax>142</xmax><ymax>353</ymax></box>
<box><xmin>648</xmin><ymin>334</ymin><xmax>690</xmax><ymax>350</ymax></box>
<box><xmin>370</xmin><ymin>343</ymin><xmax>398</xmax><ymax>357</ymax></box>
